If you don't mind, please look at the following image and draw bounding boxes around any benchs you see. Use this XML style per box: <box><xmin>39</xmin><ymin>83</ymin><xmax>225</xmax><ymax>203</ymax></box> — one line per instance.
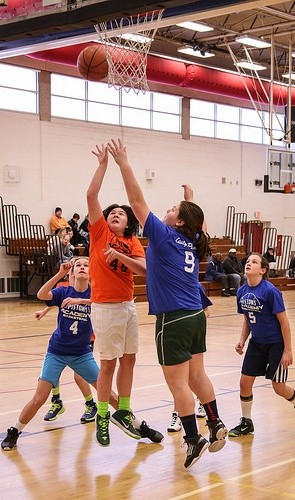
<box><xmin>8</xmin><ymin>235</ymin><xmax>295</xmax><ymax>302</ymax></box>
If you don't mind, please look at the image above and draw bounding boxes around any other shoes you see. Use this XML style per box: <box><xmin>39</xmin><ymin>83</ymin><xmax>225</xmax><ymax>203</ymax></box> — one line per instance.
<box><xmin>222</xmin><ymin>293</ymin><xmax>231</xmax><ymax>297</ymax></box>
<box><xmin>229</xmin><ymin>292</ymin><xmax>237</xmax><ymax>295</ymax></box>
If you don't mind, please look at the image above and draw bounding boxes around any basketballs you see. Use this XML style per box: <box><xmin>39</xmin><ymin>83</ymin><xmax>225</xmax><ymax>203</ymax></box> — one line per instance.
<box><xmin>77</xmin><ymin>44</ymin><xmax>108</xmax><ymax>81</ymax></box>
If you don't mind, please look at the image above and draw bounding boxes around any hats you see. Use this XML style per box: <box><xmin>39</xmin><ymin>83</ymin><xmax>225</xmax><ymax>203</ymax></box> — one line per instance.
<box><xmin>229</xmin><ymin>249</ymin><xmax>236</xmax><ymax>253</ymax></box>
<box><xmin>267</xmin><ymin>245</ymin><xmax>274</xmax><ymax>249</ymax></box>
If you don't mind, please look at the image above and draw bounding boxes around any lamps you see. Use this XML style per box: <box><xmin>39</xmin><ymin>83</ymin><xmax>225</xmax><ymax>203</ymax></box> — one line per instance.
<box><xmin>177</xmin><ymin>43</ymin><xmax>216</xmax><ymax>59</ymax></box>
<box><xmin>117</xmin><ymin>32</ymin><xmax>154</xmax><ymax>44</ymax></box>
<box><xmin>175</xmin><ymin>20</ymin><xmax>213</xmax><ymax>32</ymax></box>
<box><xmin>235</xmin><ymin>35</ymin><xmax>272</xmax><ymax>49</ymax></box>
<box><xmin>234</xmin><ymin>60</ymin><xmax>268</xmax><ymax>70</ymax></box>
<box><xmin>282</xmin><ymin>71</ymin><xmax>295</xmax><ymax>81</ymax></box>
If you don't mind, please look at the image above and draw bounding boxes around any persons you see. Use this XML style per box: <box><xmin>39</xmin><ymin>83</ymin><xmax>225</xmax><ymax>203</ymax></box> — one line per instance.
<box><xmin>288</xmin><ymin>254</ymin><xmax>295</xmax><ymax>278</ymax></box>
<box><xmin>36</xmin><ymin>255</ymin><xmax>98</xmax><ymax>423</ymax></box>
<box><xmin>1</xmin><ymin>257</ymin><xmax>166</xmax><ymax>453</ymax></box>
<box><xmin>84</xmin><ymin>143</ymin><xmax>148</xmax><ymax>446</ymax></box>
<box><xmin>104</xmin><ymin>137</ymin><xmax>227</xmax><ymax>472</ymax></box>
<box><xmin>45</xmin><ymin>206</ymin><xmax>91</xmax><ymax>279</ymax></box>
<box><xmin>225</xmin><ymin>252</ymin><xmax>295</xmax><ymax>438</ymax></box>
<box><xmin>166</xmin><ymin>283</ymin><xmax>214</xmax><ymax>433</ymax></box>
<box><xmin>205</xmin><ymin>247</ymin><xmax>280</xmax><ymax>298</ymax></box>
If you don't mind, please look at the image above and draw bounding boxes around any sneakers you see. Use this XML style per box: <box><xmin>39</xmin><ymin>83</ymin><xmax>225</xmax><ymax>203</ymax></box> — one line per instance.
<box><xmin>167</xmin><ymin>412</ymin><xmax>182</xmax><ymax>431</ymax></box>
<box><xmin>44</xmin><ymin>399</ymin><xmax>65</xmax><ymax>420</ymax></box>
<box><xmin>1</xmin><ymin>426</ymin><xmax>22</xmax><ymax>450</ymax></box>
<box><xmin>178</xmin><ymin>433</ymin><xmax>210</xmax><ymax>469</ymax></box>
<box><xmin>228</xmin><ymin>416</ymin><xmax>254</xmax><ymax>437</ymax></box>
<box><xmin>95</xmin><ymin>413</ymin><xmax>113</xmax><ymax>448</ymax></box>
<box><xmin>196</xmin><ymin>398</ymin><xmax>211</xmax><ymax>417</ymax></box>
<box><xmin>135</xmin><ymin>420</ymin><xmax>164</xmax><ymax>443</ymax></box>
<box><xmin>79</xmin><ymin>401</ymin><xmax>98</xmax><ymax>422</ymax></box>
<box><xmin>110</xmin><ymin>408</ymin><xmax>142</xmax><ymax>439</ymax></box>
<box><xmin>205</xmin><ymin>418</ymin><xmax>228</xmax><ymax>453</ymax></box>
